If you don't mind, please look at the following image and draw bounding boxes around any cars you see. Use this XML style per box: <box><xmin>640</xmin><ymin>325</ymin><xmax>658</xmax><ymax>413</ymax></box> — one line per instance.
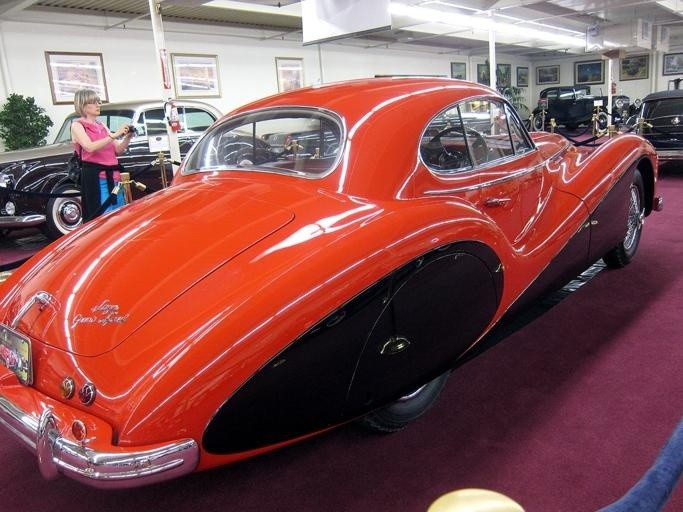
<box><xmin>634</xmin><ymin>89</ymin><xmax>683</xmax><ymax>163</ymax></box>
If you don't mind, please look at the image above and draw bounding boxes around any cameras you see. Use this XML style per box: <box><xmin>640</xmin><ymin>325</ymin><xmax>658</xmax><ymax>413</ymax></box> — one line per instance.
<box><xmin>125</xmin><ymin>125</ymin><xmax>137</xmax><ymax>133</ymax></box>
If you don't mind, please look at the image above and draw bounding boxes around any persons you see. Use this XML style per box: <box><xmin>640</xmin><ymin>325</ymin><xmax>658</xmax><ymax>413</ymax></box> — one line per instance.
<box><xmin>479</xmin><ymin>104</ymin><xmax>487</xmax><ymax>113</ymax></box>
<box><xmin>70</xmin><ymin>88</ymin><xmax>137</xmax><ymax>225</ymax></box>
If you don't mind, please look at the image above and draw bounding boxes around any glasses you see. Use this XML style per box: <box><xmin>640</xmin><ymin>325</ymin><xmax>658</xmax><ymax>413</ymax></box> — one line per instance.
<box><xmin>87</xmin><ymin>100</ymin><xmax>102</xmax><ymax>104</ymax></box>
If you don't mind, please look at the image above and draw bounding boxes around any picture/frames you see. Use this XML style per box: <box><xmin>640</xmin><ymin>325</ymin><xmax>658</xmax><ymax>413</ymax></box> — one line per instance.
<box><xmin>276</xmin><ymin>57</ymin><xmax>303</xmax><ymax>97</ymax></box>
<box><xmin>171</xmin><ymin>53</ymin><xmax>222</xmax><ymax>99</ymax></box>
<box><xmin>663</xmin><ymin>53</ymin><xmax>683</xmax><ymax>75</ymax></box>
<box><xmin>619</xmin><ymin>53</ymin><xmax>649</xmax><ymax>81</ymax></box>
<box><xmin>517</xmin><ymin>67</ymin><xmax>529</xmax><ymax>87</ymax></box>
<box><xmin>536</xmin><ymin>65</ymin><xmax>560</xmax><ymax>84</ymax></box>
<box><xmin>497</xmin><ymin>64</ymin><xmax>511</xmax><ymax>88</ymax></box>
<box><xmin>573</xmin><ymin>59</ymin><xmax>605</xmax><ymax>85</ymax></box>
<box><xmin>45</xmin><ymin>51</ymin><xmax>110</xmax><ymax>104</ymax></box>
<box><xmin>451</xmin><ymin>62</ymin><xmax>466</xmax><ymax>80</ymax></box>
<box><xmin>477</xmin><ymin>64</ymin><xmax>489</xmax><ymax>85</ymax></box>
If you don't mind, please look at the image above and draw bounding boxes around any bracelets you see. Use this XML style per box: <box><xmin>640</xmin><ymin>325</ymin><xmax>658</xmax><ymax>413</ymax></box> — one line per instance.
<box><xmin>109</xmin><ymin>134</ymin><xmax>116</xmax><ymax>140</ymax></box>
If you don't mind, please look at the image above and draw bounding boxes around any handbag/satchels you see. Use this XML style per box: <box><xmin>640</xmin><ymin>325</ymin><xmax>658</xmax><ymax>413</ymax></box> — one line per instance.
<box><xmin>66</xmin><ymin>122</ymin><xmax>85</xmax><ymax>185</ymax></box>
